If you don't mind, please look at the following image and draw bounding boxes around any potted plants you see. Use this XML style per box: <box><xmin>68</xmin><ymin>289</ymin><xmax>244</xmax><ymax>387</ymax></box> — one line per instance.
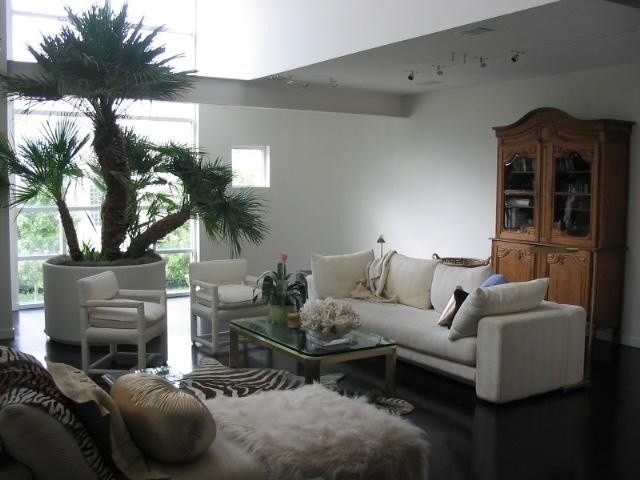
<box><xmin>0</xmin><ymin>3</ymin><xmax>270</xmax><ymax>345</ymax></box>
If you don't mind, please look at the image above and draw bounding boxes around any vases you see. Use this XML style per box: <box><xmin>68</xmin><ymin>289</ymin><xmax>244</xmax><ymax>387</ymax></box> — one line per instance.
<box><xmin>268</xmin><ymin>305</ymin><xmax>296</xmax><ymax>324</ymax></box>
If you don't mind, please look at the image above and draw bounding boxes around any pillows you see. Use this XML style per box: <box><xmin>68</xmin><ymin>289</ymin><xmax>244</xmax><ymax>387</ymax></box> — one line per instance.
<box><xmin>42</xmin><ymin>356</ymin><xmax>216</xmax><ymax>480</ymax></box>
<box><xmin>311</xmin><ymin>251</ymin><xmax>552</xmax><ymax>343</ymax></box>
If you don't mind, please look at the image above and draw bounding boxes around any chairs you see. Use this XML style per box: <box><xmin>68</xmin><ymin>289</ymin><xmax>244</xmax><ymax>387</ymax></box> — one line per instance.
<box><xmin>1</xmin><ymin>345</ymin><xmax>429</xmax><ymax>480</ymax></box>
<box><xmin>75</xmin><ymin>270</ymin><xmax>168</xmax><ymax>378</ymax></box>
<box><xmin>189</xmin><ymin>258</ymin><xmax>268</xmax><ymax>352</ymax></box>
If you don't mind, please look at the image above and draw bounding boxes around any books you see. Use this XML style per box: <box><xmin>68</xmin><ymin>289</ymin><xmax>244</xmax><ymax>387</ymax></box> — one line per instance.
<box><xmin>503</xmin><ymin>155</ymin><xmax>589</xmax><ymax>232</ymax></box>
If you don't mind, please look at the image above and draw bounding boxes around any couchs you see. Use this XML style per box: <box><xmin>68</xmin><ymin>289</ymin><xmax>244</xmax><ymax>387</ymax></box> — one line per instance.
<box><xmin>307</xmin><ymin>268</ymin><xmax>585</xmax><ymax>405</ymax></box>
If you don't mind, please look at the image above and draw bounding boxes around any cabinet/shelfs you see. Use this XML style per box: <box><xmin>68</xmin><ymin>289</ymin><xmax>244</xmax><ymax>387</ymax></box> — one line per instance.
<box><xmin>490</xmin><ymin>105</ymin><xmax>636</xmax><ymax>358</ymax></box>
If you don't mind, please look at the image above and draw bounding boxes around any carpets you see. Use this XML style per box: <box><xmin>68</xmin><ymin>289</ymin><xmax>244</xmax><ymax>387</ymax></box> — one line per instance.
<box><xmin>179</xmin><ymin>357</ymin><xmax>415</xmax><ymax>417</ymax></box>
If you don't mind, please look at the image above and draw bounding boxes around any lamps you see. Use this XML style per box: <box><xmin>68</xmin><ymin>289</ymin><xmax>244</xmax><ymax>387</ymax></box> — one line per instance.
<box><xmin>407</xmin><ymin>68</ymin><xmax>418</xmax><ymax>82</ymax></box>
<box><xmin>509</xmin><ymin>51</ymin><xmax>520</xmax><ymax>63</ymax></box>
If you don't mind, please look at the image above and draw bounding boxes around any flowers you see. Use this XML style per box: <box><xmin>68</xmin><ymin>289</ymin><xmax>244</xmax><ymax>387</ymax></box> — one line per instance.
<box><xmin>251</xmin><ymin>251</ymin><xmax>309</xmax><ymax>312</ymax></box>
<box><xmin>299</xmin><ymin>297</ymin><xmax>362</xmax><ymax>339</ymax></box>
